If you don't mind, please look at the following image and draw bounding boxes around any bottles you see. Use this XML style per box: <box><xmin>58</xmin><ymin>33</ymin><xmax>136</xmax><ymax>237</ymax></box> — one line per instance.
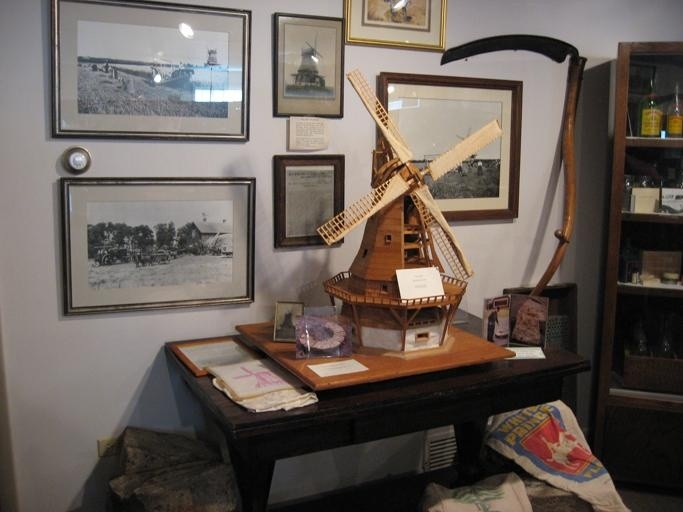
<box><xmin>638</xmin><ymin>76</ymin><xmax>683</xmax><ymax>138</ymax></box>
<box><xmin>629</xmin><ymin>310</ymin><xmax>676</xmax><ymax>358</ymax></box>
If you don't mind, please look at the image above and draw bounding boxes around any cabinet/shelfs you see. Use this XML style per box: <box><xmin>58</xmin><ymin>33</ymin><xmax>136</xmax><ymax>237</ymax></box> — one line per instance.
<box><xmin>593</xmin><ymin>41</ymin><xmax>683</xmax><ymax>497</ymax></box>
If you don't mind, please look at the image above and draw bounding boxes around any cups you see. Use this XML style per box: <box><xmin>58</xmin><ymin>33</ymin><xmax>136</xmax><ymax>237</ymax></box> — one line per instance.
<box><xmin>621</xmin><ymin>173</ymin><xmax>664</xmax><ymax>212</ymax></box>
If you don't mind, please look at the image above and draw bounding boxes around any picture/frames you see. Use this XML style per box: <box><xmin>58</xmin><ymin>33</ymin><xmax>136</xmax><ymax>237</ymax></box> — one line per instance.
<box><xmin>60</xmin><ymin>177</ymin><xmax>258</xmax><ymax>317</ymax></box>
<box><xmin>50</xmin><ymin>0</ymin><xmax>252</xmax><ymax>143</ymax></box>
<box><xmin>273</xmin><ymin>12</ymin><xmax>346</xmax><ymax>120</ymax></box>
<box><xmin>273</xmin><ymin>155</ymin><xmax>347</xmax><ymax>248</ymax></box>
<box><xmin>380</xmin><ymin>72</ymin><xmax>523</xmax><ymax>221</ymax></box>
<box><xmin>273</xmin><ymin>302</ymin><xmax>306</xmax><ymax>343</ymax></box>
<box><xmin>342</xmin><ymin>1</ymin><xmax>448</xmax><ymax>53</ymax></box>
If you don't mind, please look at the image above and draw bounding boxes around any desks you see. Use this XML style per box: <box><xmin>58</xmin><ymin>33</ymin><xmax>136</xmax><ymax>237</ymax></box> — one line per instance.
<box><xmin>167</xmin><ymin>308</ymin><xmax>591</xmax><ymax>512</ymax></box>
